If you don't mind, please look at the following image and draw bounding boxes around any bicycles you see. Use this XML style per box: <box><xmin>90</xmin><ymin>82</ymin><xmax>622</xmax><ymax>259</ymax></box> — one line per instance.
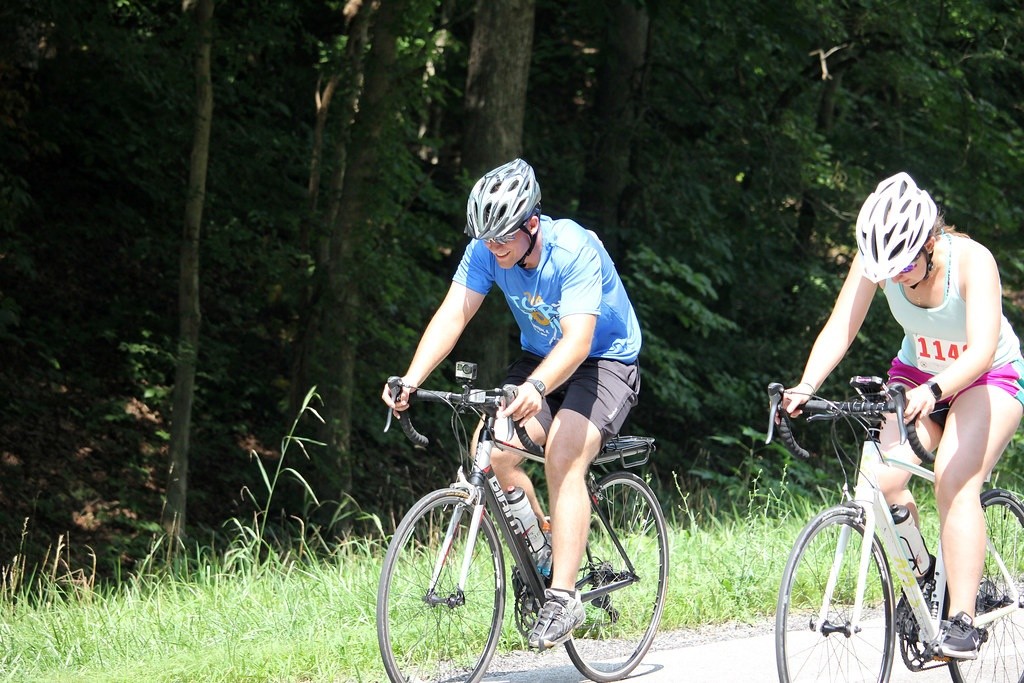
<box><xmin>376</xmin><ymin>361</ymin><xmax>670</xmax><ymax>683</ymax></box>
<box><xmin>765</xmin><ymin>375</ymin><xmax>1024</xmax><ymax>683</ymax></box>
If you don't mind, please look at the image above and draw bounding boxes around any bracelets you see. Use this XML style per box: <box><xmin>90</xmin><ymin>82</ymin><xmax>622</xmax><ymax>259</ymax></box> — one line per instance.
<box><xmin>800</xmin><ymin>381</ymin><xmax>816</xmax><ymax>394</ymax></box>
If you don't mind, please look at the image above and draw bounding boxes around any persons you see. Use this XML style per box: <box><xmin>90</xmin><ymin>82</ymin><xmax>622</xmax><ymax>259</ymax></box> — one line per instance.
<box><xmin>381</xmin><ymin>157</ymin><xmax>641</xmax><ymax>649</ymax></box>
<box><xmin>770</xmin><ymin>173</ymin><xmax>1024</xmax><ymax>659</ymax></box>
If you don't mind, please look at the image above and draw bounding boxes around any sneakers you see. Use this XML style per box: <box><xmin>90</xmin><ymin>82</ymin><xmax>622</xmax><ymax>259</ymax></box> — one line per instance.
<box><xmin>937</xmin><ymin>611</ymin><xmax>980</xmax><ymax>660</ymax></box>
<box><xmin>895</xmin><ymin>553</ymin><xmax>937</xmax><ymax>636</ymax></box>
<box><xmin>526</xmin><ymin>588</ymin><xmax>586</xmax><ymax>653</ymax></box>
<box><xmin>526</xmin><ymin>520</ymin><xmax>554</xmax><ymax>611</ymax></box>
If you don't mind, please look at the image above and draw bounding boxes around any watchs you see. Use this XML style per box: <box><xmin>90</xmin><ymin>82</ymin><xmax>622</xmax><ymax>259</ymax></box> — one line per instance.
<box><xmin>526</xmin><ymin>378</ymin><xmax>547</xmax><ymax>401</ymax></box>
<box><xmin>922</xmin><ymin>380</ymin><xmax>944</xmax><ymax>405</ymax></box>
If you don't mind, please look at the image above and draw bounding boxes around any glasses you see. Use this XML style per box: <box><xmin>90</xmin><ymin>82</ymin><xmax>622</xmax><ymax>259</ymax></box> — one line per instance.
<box><xmin>899</xmin><ymin>231</ymin><xmax>933</xmax><ymax>276</ymax></box>
<box><xmin>483</xmin><ymin>212</ymin><xmax>536</xmax><ymax>245</ymax></box>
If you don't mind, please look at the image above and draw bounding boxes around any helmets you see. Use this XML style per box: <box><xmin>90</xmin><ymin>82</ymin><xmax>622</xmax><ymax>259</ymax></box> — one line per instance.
<box><xmin>463</xmin><ymin>158</ymin><xmax>541</xmax><ymax>244</ymax></box>
<box><xmin>855</xmin><ymin>171</ymin><xmax>937</xmax><ymax>284</ymax></box>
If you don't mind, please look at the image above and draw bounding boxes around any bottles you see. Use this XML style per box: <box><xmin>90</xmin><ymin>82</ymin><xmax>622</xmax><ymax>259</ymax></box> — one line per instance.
<box><xmin>889</xmin><ymin>504</ymin><xmax>930</xmax><ymax>577</ymax></box>
<box><xmin>538</xmin><ymin>516</ymin><xmax>553</xmax><ymax>576</ymax></box>
<box><xmin>504</xmin><ymin>485</ymin><xmax>544</xmax><ymax>552</ymax></box>
